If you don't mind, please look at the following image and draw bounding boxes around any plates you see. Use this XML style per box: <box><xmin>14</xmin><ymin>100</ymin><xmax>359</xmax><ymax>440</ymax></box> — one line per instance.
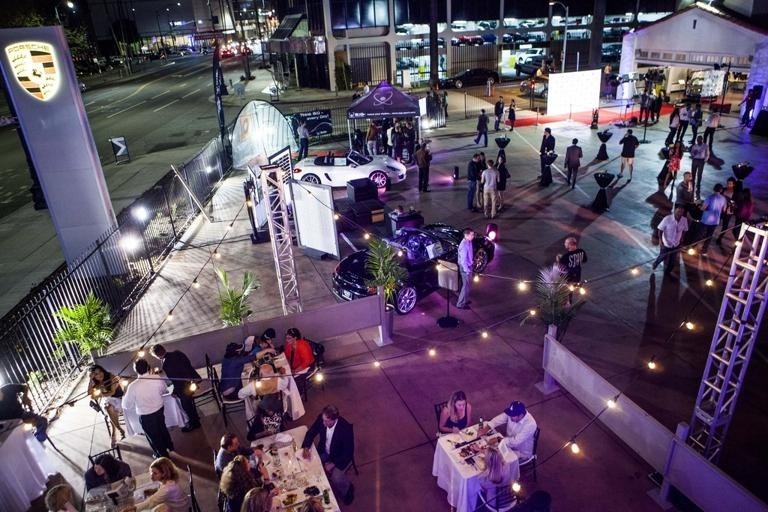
<box><xmin>269</xmin><ymin>432</ymin><xmax>334</xmax><ymax>512</ymax></box>
<box><xmin>447</xmin><ymin>428</ymin><xmax>504</xmax><ymax>470</ymax></box>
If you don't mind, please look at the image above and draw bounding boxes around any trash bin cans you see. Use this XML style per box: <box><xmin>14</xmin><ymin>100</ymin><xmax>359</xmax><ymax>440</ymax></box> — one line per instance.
<box><xmin>234</xmin><ymin>83</ymin><xmax>244</xmax><ymax>95</ymax></box>
<box><xmin>487</xmin><ymin>81</ymin><xmax>494</xmax><ymax>97</ymax></box>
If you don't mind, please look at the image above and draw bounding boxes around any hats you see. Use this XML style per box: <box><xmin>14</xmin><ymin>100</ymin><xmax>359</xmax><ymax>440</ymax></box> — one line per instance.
<box><xmin>260</xmin><ymin>328</ymin><xmax>275</xmax><ymax>340</ymax></box>
<box><xmin>225</xmin><ymin>342</ymin><xmax>245</xmax><ymax>352</ymax></box>
<box><xmin>503</xmin><ymin>400</ymin><xmax>525</xmax><ymax>417</ymax></box>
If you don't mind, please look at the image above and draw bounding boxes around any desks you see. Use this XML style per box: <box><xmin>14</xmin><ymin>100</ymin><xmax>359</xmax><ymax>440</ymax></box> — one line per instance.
<box><xmin>2</xmin><ymin>419</ymin><xmax>49</xmax><ymax>511</ymax></box>
<box><xmin>593</xmin><ymin>171</ymin><xmax>616</xmax><ymax>212</ymax></box>
<box><xmin>539</xmin><ymin>152</ymin><xmax>558</xmax><ymax>185</ymax></box>
<box><xmin>729</xmin><ymin>162</ymin><xmax>756</xmax><ymax>193</ymax></box>
<box><xmin>595</xmin><ymin>130</ymin><xmax>614</xmax><ymax>161</ymax></box>
<box><xmin>655</xmin><ymin>147</ymin><xmax>671</xmax><ymax>181</ymax></box>
<box><xmin>494</xmin><ymin>137</ymin><xmax>512</xmax><ymax>163</ymax></box>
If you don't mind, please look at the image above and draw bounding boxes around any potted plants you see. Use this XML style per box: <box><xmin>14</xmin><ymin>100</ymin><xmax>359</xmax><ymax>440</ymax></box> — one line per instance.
<box><xmin>363</xmin><ymin>235</ymin><xmax>409</xmax><ymax>338</ymax></box>
<box><xmin>49</xmin><ymin>290</ymin><xmax>113</xmax><ymax>366</ymax></box>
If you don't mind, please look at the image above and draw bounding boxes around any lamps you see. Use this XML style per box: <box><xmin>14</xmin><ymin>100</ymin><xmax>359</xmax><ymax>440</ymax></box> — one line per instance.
<box><xmin>568</xmin><ymin>437</ymin><xmax>581</xmax><ymax>454</ymax></box>
<box><xmin>568</xmin><ymin>280</ymin><xmax>589</xmax><ymax>295</ymax></box>
<box><xmin>648</xmin><ymin>354</ymin><xmax>660</xmax><ymax>370</ymax></box>
<box><xmin>685</xmin><ymin>313</ymin><xmax>697</xmax><ymax>329</ymax></box>
<box><xmin>607</xmin><ymin>394</ymin><xmax>619</xmax><ymax>409</ymax></box>
<box><xmin>702</xmin><ymin>271</ymin><xmax>713</xmax><ymax>286</ymax></box>
<box><xmin>687</xmin><ymin>244</ymin><xmax>696</xmax><ymax>255</ymax></box>
<box><xmin>630</xmin><ymin>263</ymin><xmax>641</xmax><ymax>276</ymax></box>
<box><xmin>735</xmin><ymin>234</ymin><xmax>745</xmax><ymax>247</ymax></box>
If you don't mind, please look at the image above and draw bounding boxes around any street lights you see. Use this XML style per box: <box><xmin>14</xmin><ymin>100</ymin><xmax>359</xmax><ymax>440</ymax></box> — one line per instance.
<box><xmin>546</xmin><ymin>0</ymin><xmax>569</xmax><ymax>73</ymax></box>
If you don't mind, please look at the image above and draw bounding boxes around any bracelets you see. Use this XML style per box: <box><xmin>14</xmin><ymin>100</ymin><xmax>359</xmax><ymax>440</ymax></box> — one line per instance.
<box><xmin>263</xmin><ymin>477</ymin><xmax>270</xmax><ymax>481</ymax></box>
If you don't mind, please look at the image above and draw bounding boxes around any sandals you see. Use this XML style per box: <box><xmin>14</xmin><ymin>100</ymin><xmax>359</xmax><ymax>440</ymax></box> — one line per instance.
<box><xmin>109</xmin><ymin>430</ymin><xmax>126</xmax><ymax>449</ymax></box>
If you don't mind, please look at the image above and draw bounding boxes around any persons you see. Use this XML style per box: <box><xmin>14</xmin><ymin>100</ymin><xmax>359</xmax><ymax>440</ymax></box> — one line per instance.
<box><xmin>478</xmin><ymin>400</ymin><xmax>537</xmax><ymax>467</ymax></box>
<box><xmin>217</xmin><ymin>327</ymin><xmax>317</xmax><ymax>415</ymax></box>
<box><xmin>558</xmin><ymin>236</ymin><xmax>587</xmax><ymax>307</ymax></box>
<box><xmin>44</xmin><ymin>483</ymin><xmax>80</xmax><ymax>512</ymax></box>
<box><xmin>219</xmin><ymin>453</ymin><xmax>279</xmax><ymax>512</ymax></box>
<box><xmin>128</xmin><ymin>456</ymin><xmax>190</xmax><ymax>512</ymax></box>
<box><xmin>0</xmin><ymin>383</ymin><xmax>49</xmax><ymax>449</ymax></box>
<box><xmin>87</xmin><ymin>363</ymin><xmax>125</xmax><ymax>451</ymax></box>
<box><xmin>300</xmin><ymin>498</ymin><xmax>324</xmax><ymax>511</ymax></box>
<box><xmin>297</xmin><ymin>120</ymin><xmax>309</xmax><ymax>161</ymax></box>
<box><xmin>85</xmin><ymin>454</ymin><xmax>132</xmax><ymax>493</ymax></box>
<box><xmin>246</xmin><ymin>395</ymin><xmax>290</xmax><ymax>440</ymax></box>
<box><xmin>456</xmin><ymin>227</ymin><xmax>475</xmax><ymax>309</ymax></box>
<box><xmin>239</xmin><ymin>486</ymin><xmax>273</xmax><ymax>512</ymax></box>
<box><xmin>214</xmin><ymin>432</ymin><xmax>265</xmax><ymax>482</ymax></box>
<box><xmin>149</xmin><ymin>343</ymin><xmax>203</xmax><ymax>432</ymax></box>
<box><xmin>121</xmin><ymin>357</ymin><xmax>176</xmax><ymax>459</ymax></box>
<box><xmin>300</xmin><ymin>404</ymin><xmax>356</xmax><ymax>505</ymax></box>
<box><xmin>438</xmin><ymin>389</ymin><xmax>471</xmax><ymax>435</ymax></box>
<box><xmin>477</xmin><ymin>447</ymin><xmax>518</xmax><ymax>511</ymax></box>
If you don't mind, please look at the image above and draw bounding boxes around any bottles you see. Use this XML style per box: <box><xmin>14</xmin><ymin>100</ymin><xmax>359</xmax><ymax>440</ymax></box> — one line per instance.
<box><xmin>479</xmin><ymin>416</ymin><xmax>483</xmax><ymax>430</ymax></box>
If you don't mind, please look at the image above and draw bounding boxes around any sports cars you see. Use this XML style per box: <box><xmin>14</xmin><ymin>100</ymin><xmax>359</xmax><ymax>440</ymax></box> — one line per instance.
<box><xmin>325</xmin><ymin>220</ymin><xmax>498</xmax><ymax>321</ymax></box>
<box><xmin>291</xmin><ymin>148</ymin><xmax>409</xmax><ymax>188</ymax></box>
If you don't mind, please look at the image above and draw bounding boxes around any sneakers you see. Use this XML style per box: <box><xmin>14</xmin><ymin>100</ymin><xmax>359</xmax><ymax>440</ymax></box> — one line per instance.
<box><xmin>344</xmin><ymin>483</ymin><xmax>354</xmax><ymax>505</ymax></box>
<box><xmin>152</xmin><ymin>441</ymin><xmax>175</xmax><ymax>460</ymax></box>
<box><xmin>419</xmin><ymin>183</ymin><xmax>431</xmax><ymax>192</ymax></box>
<box><xmin>467</xmin><ymin>202</ymin><xmax>503</xmax><ymax>218</ymax></box>
<box><xmin>457</xmin><ymin>300</ymin><xmax>471</xmax><ymax>310</ymax></box>
<box><xmin>181</xmin><ymin>422</ymin><xmax>201</xmax><ymax>432</ymax></box>
<box><xmin>617</xmin><ymin>173</ymin><xmax>632</xmax><ymax>181</ymax></box>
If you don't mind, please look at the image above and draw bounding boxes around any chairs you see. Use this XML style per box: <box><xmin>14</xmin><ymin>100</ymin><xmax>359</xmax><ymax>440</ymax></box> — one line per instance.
<box><xmin>433</xmin><ymin>401</ymin><xmax>449</xmax><ymax>435</ymax></box>
<box><xmin>518</xmin><ymin>425</ymin><xmax>542</xmax><ymax>484</ymax></box>
<box><xmin>21</xmin><ymin>402</ymin><xmax>57</xmax><ymax>450</ymax></box>
<box><xmin>89</xmin><ymin>342</ymin><xmax>323</xmax><ymax>429</ymax></box>
<box><xmin>84</xmin><ymin>416</ymin><xmax>359</xmax><ymax>512</ymax></box>
<box><xmin>475</xmin><ymin>478</ymin><xmax>520</xmax><ymax>511</ymax></box>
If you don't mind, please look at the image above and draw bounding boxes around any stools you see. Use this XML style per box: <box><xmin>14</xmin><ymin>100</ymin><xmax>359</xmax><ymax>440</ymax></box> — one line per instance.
<box><xmin>709</xmin><ymin>101</ymin><xmax>733</xmax><ymax>115</ymax></box>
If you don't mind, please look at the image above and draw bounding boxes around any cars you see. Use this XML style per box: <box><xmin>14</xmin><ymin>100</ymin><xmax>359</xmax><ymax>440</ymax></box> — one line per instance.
<box><xmin>199</xmin><ymin>45</ymin><xmax>215</xmax><ymax>55</ymax></box>
<box><xmin>97</xmin><ymin>44</ymin><xmax>193</xmax><ymax>66</ymax></box>
<box><xmin>385</xmin><ymin>10</ymin><xmax>636</xmax><ymax>95</ymax></box>
<box><xmin>308</xmin><ymin>121</ymin><xmax>332</xmax><ymax>135</ymax></box>
<box><xmin>220</xmin><ymin>36</ymin><xmax>260</xmax><ymax>58</ymax></box>
